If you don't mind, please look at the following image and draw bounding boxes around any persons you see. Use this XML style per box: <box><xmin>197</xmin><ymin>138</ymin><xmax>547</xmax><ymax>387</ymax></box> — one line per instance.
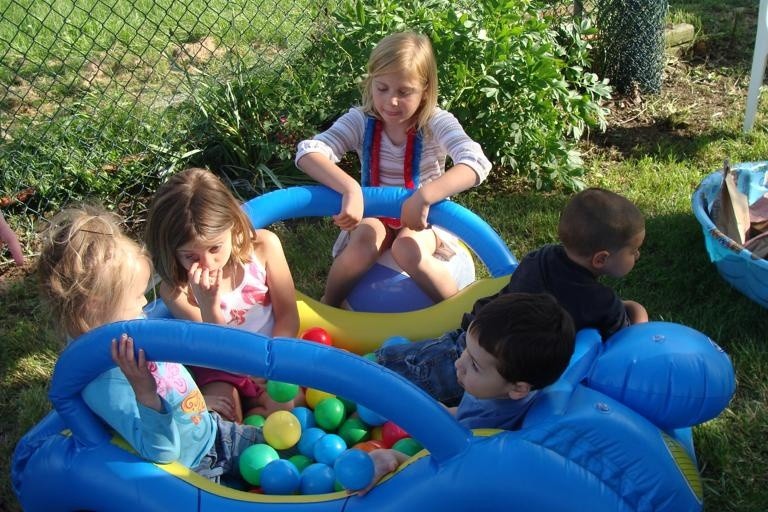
<box><xmin>294</xmin><ymin>32</ymin><xmax>491</xmax><ymax>307</ymax></box>
<box><xmin>344</xmin><ymin>292</ymin><xmax>577</xmax><ymax>499</ymax></box>
<box><xmin>375</xmin><ymin>188</ymin><xmax>648</xmax><ymax>405</ymax></box>
<box><xmin>0</xmin><ymin>209</ymin><xmax>23</xmax><ymax>265</ymax></box>
<box><xmin>142</xmin><ymin>167</ymin><xmax>299</xmax><ymax>425</ymax></box>
<box><xmin>34</xmin><ymin>194</ymin><xmax>300</xmax><ymax>493</ymax></box>
